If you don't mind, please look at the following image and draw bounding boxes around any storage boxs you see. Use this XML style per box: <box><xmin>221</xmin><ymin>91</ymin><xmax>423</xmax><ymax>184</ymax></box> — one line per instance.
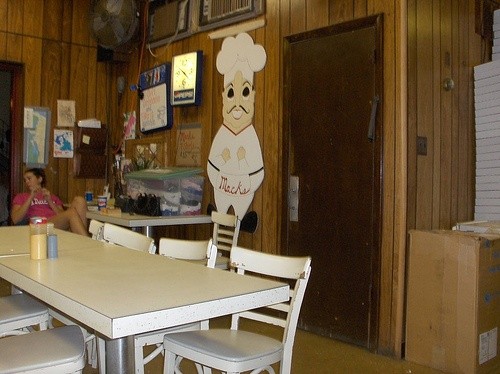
<box><xmin>124</xmin><ymin>166</ymin><xmax>204</xmax><ymax>216</ymax></box>
<box><xmin>407</xmin><ymin>229</ymin><xmax>500</xmax><ymax>374</ymax></box>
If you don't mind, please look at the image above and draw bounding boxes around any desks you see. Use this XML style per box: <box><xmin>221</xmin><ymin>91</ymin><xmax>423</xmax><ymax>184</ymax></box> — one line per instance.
<box><xmin>0</xmin><ymin>226</ymin><xmax>100</xmax><ymax>296</ymax></box>
<box><xmin>0</xmin><ymin>244</ymin><xmax>290</xmax><ymax>374</ymax></box>
<box><xmin>86</xmin><ymin>209</ymin><xmax>211</xmax><ymax>238</ymax></box>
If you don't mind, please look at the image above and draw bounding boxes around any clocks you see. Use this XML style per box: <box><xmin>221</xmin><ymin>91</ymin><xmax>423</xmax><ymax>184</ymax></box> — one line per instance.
<box><xmin>170</xmin><ymin>49</ymin><xmax>203</xmax><ymax>107</ymax></box>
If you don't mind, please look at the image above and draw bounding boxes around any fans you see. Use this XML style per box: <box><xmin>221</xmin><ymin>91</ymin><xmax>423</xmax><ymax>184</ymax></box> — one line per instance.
<box><xmin>86</xmin><ymin>0</ymin><xmax>142</xmax><ymax>54</ymax></box>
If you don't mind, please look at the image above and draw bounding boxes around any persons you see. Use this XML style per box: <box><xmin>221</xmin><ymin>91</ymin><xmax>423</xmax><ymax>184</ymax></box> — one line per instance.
<box><xmin>10</xmin><ymin>168</ymin><xmax>91</xmax><ymax>237</ymax></box>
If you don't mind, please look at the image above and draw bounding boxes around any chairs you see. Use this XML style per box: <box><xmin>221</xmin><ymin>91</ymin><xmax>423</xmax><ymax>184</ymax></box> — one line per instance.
<box><xmin>0</xmin><ymin>220</ymin><xmax>311</xmax><ymax>374</ymax></box>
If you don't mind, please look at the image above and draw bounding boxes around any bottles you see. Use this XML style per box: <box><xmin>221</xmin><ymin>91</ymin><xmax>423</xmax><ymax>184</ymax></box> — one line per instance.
<box><xmin>85</xmin><ymin>192</ymin><xmax>93</xmax><ymax>201</ymax></box>
<box><xmin>46</xmin><ymin>233</ymin><xmax>58</xmax><ymax>258</ymax></box>
<box><xmin>29</xmin><ymin>217</ymin><xmax>48</xmax><ymax>260</ymax></box>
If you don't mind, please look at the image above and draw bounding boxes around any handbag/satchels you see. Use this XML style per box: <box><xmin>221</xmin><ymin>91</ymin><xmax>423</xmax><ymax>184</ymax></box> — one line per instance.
<box><xmin>134</xmin><ymin>193</ymin><xmax>162</xmax><ymax>217</ymax></box>
<box><xmin>114</xmin><ymin>193</ymin><xmax>132</xmax><ymax>213</ymax></box>
